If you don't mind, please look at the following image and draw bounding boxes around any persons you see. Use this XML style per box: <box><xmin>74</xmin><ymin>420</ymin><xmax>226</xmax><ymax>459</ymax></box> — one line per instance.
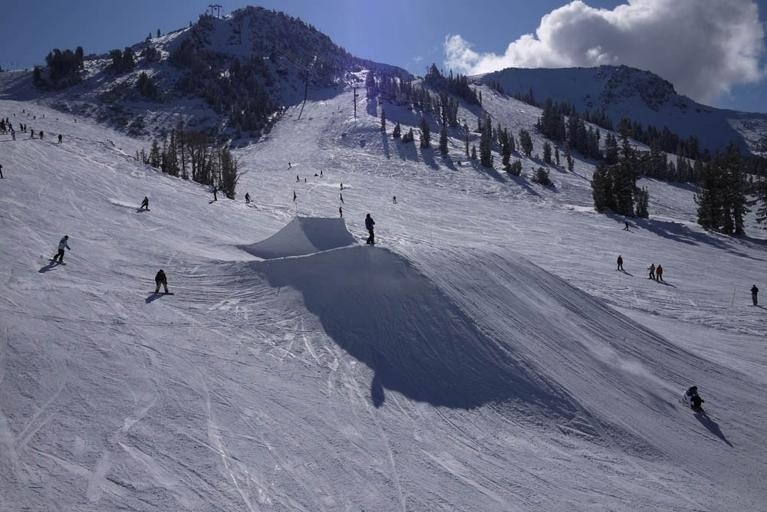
<box><xmin>245</xmin><ymin>193</ymin><xmax>249</xmax><ymax>201</ymax></box>
<box><xmin>648</xmin><ymin>264</ymin><xmax>655</xmax><ymax>280</ymax></box>
<box><xmin>155</xmin><ymin>270</ymin><xmax>168</xmax><ymax>294</ymax></box>
<box><xmin>141</xmin><ymin>197</ymin><xmax>148</xmax><ymax>209</ymax></box>
<box><xmin>214</xmin><ymin>187</ymin><xmax>217</xmax><ymax>200</ymax></box>
<box><xmin>0</xmin><ymin>110</ymin><xmax>77</xmax><ymax>142</ymax></box>
<box><xmin>656</xmin><ymin>264</ymin><xmax>663</xmax><ymax>281</ymax></box>
<box><xmin>617</xmin><ymin>255</ymin><xmax>623</xmax><ymax>270</ymax></box>
<box><xmin>625</xmin><ymin>223</ymin><xmax>628</xmax><ymax>231</ymax></box>
<box><xmin>288</xmin><ymin>160</ymin><xmax>396</xmax><ymax>245</ymax></box>
<box><xmin>0</xmin><ymin>164</ymin><xmax>3</xmax><ymax>179</ymax></box>
<box><xmin>680</xmin><ymin>386</ymin><xmax>703</xmax><ymax>408</ymax></box>
<box><xmin>55</xmin><ymin>236</ymin><xmax>70</xmax><ymax>262</ymax></box>
<box><xmin>752</xmin><ymin>285</ymin><xmax>759</xmax><ymax>305</ymax></box>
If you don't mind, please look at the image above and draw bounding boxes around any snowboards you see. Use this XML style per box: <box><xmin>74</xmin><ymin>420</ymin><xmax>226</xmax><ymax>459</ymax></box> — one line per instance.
<box><xmin>149</xmin><ymin>291</ymin><xmax>174</xmax><ymax>296</ymax></box>
<box><xmin>48</xmin><ymin>257</ymin><xmax>69</xmax><ymax>265</ymax></box>
